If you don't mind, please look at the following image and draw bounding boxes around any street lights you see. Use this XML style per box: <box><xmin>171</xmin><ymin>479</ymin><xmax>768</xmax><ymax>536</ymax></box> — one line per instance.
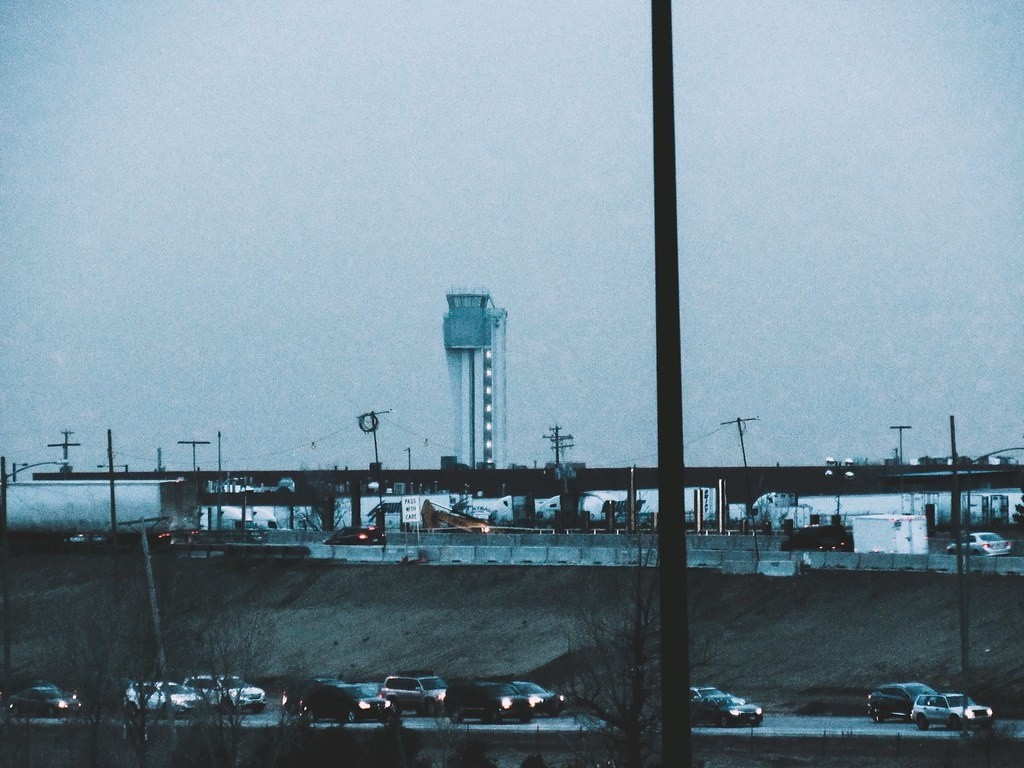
<box><xmin>0</xmin><ymin>456</ymin><xmax>69</xmax><ymax>696</ymax></box>
<box><xmin>889</xmin><ymin>426</ymin><xmax>912</xmax><ymax>492</ymax></box>
<box><xmin>97</xmin><ymin>464</ymin><xmax>130</xmax><ymax>472</ymax></box>
<box><xmin>177</xmin><ymin>439</ymin><xmax>210</xmax><ymax>498</ymax></box>
<box><xmin>824</xmin><ymin>456</ymin><xmax>855</xmax><ymax>526</ymax></box>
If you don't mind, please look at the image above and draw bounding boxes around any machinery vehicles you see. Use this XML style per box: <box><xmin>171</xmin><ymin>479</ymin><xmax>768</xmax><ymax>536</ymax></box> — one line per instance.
<box><xmin>422</xmin><ymin>499</ymin><xmax>491</xmax><ymax>533</ymax></box>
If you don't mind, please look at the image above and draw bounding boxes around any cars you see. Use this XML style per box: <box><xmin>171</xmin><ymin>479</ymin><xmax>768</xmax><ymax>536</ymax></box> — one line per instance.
<box><xmin>9</xmin><ymin>686</ymin><xmax>82</xmax><ymax>718</ymax></box>
<box><xmin>690</xmin><ymin>686</ymin><xmax>762</xmax><ymax>728</ymax></box>
<box><xmin>282</xmin><ymin>678</ymin><xmax>390</xmax><ymax>723</ymax></box>
<box><xmin>509</xmin><ymin>681</ymin><xmax>566</xmax><ymax>716</ymax></box>
<box><xmin>945</xmin><ymin>532</ymin><xmax>1012</xmax><ymax>556</ymax></box>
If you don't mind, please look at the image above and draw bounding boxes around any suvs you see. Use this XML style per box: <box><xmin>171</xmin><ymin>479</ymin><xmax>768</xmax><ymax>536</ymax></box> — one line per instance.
<box><xmin>443</xmin><ymin>680</ymin><xmax>535</xmax><ymax>723</ymax></box>
<box><xmin>377</xmin><ymin>671</ymin><xmax>448</xmax><ymax>717</ymax></box>
<box><xmin>867</xmin><ymin>683</ymin><xmax>939</xmax><ymax>723</ymax></box>
<box><xmin>910</xmin><ymin>691</ymin><xmax>994</xmax><ymax>730</ymax></box>
<box><xmin>124</xmin><ymin>681</ymin><xmax>206</xmax><ymax>717</ymax></box>
<box><xmin>323</xmin><ymin>527</ymin><xmax>386</xmax><ymax>545</ymax></box>
<box><xmin>183</xmin><ymin>674</ymin><xmax>266</xmax><ymax>714</ymax></box>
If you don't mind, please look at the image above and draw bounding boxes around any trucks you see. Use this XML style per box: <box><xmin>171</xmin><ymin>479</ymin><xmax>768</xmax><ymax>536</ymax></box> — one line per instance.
<box><xmin>780</xmin><ymin>514</ymin><xmax>930</xmax><ymax>554</ymax></box>
